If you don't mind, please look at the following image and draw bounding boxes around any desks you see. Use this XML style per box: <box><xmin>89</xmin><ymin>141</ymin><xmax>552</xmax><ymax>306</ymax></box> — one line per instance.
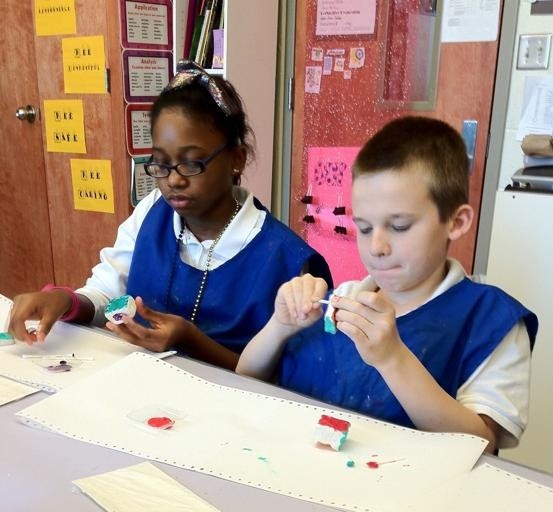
<box><xmin>0</xmin><ymin>325</ymin><xmax>553</xmax><ymax>511</ymax></box>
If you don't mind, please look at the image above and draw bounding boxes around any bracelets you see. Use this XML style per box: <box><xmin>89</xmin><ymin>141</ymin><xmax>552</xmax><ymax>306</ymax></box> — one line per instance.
<box><xmin>39</xmin><ymin>283</ymin><xmax>80</xmax><ymax>322</ymax></box>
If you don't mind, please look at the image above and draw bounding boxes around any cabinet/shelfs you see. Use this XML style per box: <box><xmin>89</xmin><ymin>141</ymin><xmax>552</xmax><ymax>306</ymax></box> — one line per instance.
<box><xmin>172</xmin><ymin>0</ymin><xmax>279</xmax><ymax>214</ymax></box>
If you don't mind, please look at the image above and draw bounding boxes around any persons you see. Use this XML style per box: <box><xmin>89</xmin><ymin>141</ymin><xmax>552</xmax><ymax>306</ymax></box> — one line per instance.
<box><xmin>235</xmin><ymin>116</ymin><xmax>539</xmax><ymax>456</ymax></box>
<box><xmin>8</xmin><ymin>58</ymin><xmax>333</xmax><ymax>371</ymax></box>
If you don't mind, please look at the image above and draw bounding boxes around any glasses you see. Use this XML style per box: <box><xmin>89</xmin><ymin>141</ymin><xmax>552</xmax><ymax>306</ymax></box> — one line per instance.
<box><xmin>143</xmin><ymin>143</ymin><xmax>230</xmax><ymax>179</ymax></box>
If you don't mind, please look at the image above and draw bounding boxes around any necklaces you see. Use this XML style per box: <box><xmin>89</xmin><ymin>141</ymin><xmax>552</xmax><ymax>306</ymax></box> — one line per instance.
<box><xmin>165</xmin><ymin>197</ymin><xmax>238</xmax><ymax>323</ymax></box>
